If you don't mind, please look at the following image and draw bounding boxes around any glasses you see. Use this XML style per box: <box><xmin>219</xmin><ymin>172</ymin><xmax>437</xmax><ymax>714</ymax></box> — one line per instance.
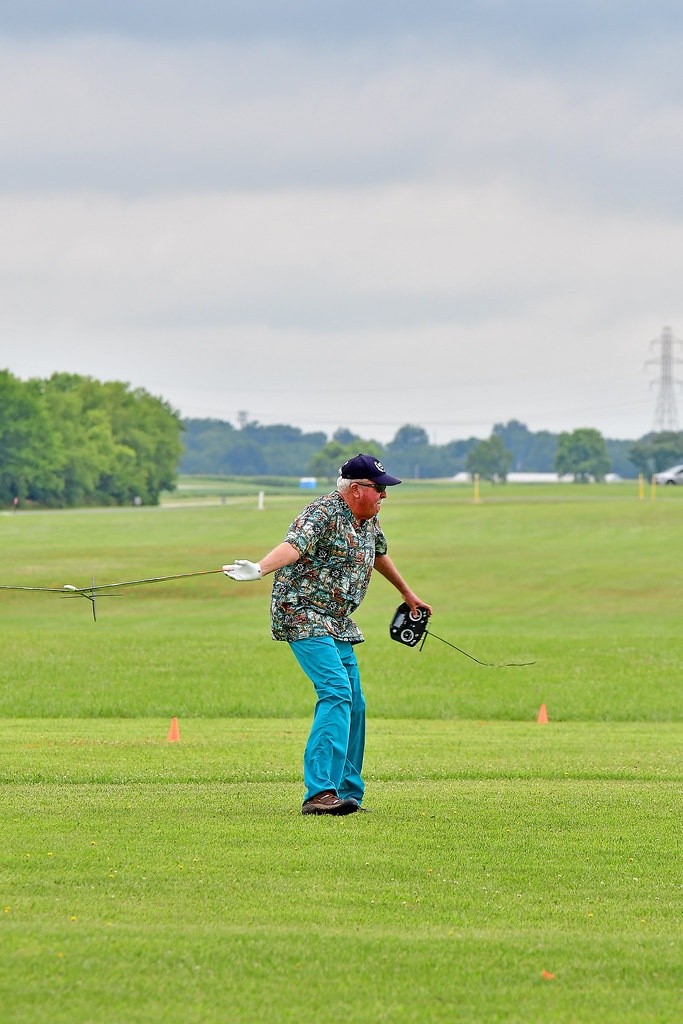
<box><xmin>358</xmin><ymin>483</ymin><xmax>386</xmax><ymax>493</ymax></box>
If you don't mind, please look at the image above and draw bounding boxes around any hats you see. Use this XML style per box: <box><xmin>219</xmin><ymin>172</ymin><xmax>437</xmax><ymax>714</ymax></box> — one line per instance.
<box><xmin>339</xmin><ymin>453</ymin><xmax>402</xmax><ymax>486</ymax></box>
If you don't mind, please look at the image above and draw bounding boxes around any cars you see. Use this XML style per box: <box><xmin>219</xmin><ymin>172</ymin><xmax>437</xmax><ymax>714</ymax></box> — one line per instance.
<box><xmin>651</xmin><ymin>464</ymin><xmax>683</xmax><ymax>485</ymax></box>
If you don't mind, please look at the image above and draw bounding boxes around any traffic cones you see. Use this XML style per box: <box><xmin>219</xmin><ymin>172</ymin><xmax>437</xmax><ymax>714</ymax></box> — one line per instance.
<box><xmin>536</xmin><ymin>704</ymin><xmax>549</xmax><ymax>724</ymax></box>
<box><xmin>168</xmin><ymin>716</ymin><xmax>181</xmax><ymax>741</ymax></box>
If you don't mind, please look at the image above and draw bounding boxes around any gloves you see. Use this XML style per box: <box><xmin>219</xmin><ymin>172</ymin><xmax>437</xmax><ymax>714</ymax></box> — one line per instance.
<box><xmin>223</xmin><ymin>560</ymin><xmax>262</xmax><ymax>581</ymax></box>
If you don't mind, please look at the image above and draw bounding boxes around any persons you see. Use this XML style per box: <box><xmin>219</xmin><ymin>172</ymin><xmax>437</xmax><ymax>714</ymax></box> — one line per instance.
<box><xmin>223</xmin><ymin>454</ymin><xmax>434</xmax><ymax>816</ymax></box>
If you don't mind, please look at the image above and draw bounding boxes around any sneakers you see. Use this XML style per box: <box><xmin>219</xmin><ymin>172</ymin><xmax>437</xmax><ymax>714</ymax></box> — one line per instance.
<box><xmin>301</xmin><ymin>790</ymin><xmax>358</xmax><ymax>816</ymax></box>
